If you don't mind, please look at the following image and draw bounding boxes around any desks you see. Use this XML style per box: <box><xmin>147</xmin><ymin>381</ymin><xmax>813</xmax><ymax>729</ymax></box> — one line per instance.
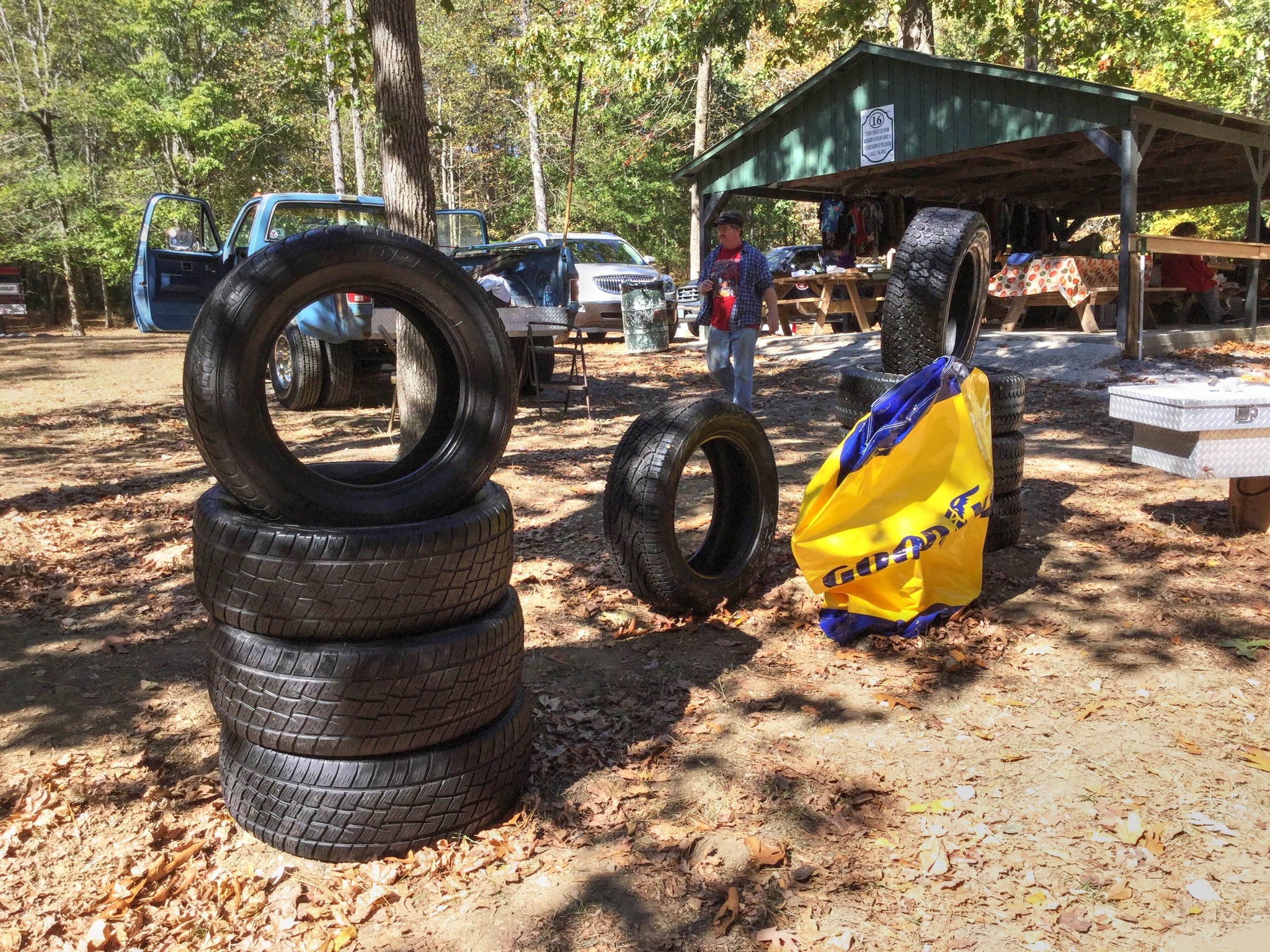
<box><xmin>773</xmin><ymin>269</ymin><xmax>890</xmax><ymax>336</ymax></box>
<box><xmin>994</xmin><ymin>256</ymin><xmax>1157</xmax><ymax>333</ymax></box>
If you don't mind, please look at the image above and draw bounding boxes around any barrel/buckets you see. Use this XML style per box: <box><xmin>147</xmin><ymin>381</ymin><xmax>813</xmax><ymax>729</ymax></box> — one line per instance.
<box><xmin>619</xmin><ymin>279</ymin><xmax>670</xmax><ymax>353</ymax></box>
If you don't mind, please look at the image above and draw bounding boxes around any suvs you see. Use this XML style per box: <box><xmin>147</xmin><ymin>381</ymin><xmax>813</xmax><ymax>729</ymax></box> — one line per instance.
<box><xmin>503</xmin><ymin>230</ymin><xmax>680</xmax><ymax>341</ymax></box>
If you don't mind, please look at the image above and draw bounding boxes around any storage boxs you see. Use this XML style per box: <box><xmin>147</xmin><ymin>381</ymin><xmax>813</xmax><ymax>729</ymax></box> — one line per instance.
<box><xmin>1108</xmin><ymin>381</ymin><xmax>1270</xmax><ymax>480</ymax></box>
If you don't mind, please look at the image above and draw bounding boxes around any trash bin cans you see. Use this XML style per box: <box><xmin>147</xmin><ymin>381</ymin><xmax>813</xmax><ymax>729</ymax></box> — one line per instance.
<box><xmin>620</xmin><ymin>279</ymin><xmax>671</xmax><ymax>354</ymax></box>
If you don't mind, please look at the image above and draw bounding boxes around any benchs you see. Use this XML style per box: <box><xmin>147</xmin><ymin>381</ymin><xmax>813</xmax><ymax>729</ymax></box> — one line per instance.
<box><xmin>762</xmin><ymin>297</ymin><xmax>886</xmax><ymax>336</ymax></box>
<box><xmin>1082</xmin><ymin>287</ymin><xmax>1187</xmax><ymax>333</ymax></box>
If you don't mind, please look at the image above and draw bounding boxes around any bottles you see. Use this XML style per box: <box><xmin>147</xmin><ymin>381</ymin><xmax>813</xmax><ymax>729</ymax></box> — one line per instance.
<box><xmin>544</xmin><ymin>282</ymin><xmax>553</xmax><ymax>307</ymax></box>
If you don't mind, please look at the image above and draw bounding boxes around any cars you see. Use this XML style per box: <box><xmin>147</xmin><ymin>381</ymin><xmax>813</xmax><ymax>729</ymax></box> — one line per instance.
<box><xmin>677</xmin><ymin>245</ymin><xmax>827</xmax><ymax>337</ymax></box>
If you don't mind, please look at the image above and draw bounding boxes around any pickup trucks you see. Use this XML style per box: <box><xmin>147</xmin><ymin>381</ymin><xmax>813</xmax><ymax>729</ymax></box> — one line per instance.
<box><xmin>131</xmin><ymin>190</ymin><xmax>580</xmax><ymax>412</ymax></box>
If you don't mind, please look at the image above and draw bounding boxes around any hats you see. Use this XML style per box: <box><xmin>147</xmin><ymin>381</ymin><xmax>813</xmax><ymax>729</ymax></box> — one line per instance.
<box><xmin>710</xmin><ymin>216</ymin><xmax>743</xmax><ymax>229</ymax></box>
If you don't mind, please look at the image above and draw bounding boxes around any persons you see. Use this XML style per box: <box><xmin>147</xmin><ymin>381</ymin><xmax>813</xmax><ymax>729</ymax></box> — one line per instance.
<box><xmin>1160</xmin><ymin>222</ymin><xmax>1226</xmax><ymax>324</ymax></box>
<box><xmin>693</xmin><ymin>210</ymin><xmax>779</xmax><ymax>411</ymax></box>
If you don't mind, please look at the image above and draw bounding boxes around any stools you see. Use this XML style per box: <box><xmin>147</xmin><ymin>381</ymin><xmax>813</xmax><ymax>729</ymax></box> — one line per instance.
<box><xmin>517</xmin><ymin>321</ymin><xmax>591</xmax><ymax>423</ymax></box>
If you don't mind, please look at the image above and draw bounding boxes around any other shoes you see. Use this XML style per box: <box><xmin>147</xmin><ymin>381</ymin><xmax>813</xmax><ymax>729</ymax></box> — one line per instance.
<box><xmin>1223</xmin><ymin>315</ymin><xmax>1244</xmax><ymax>325</ymax></box>
